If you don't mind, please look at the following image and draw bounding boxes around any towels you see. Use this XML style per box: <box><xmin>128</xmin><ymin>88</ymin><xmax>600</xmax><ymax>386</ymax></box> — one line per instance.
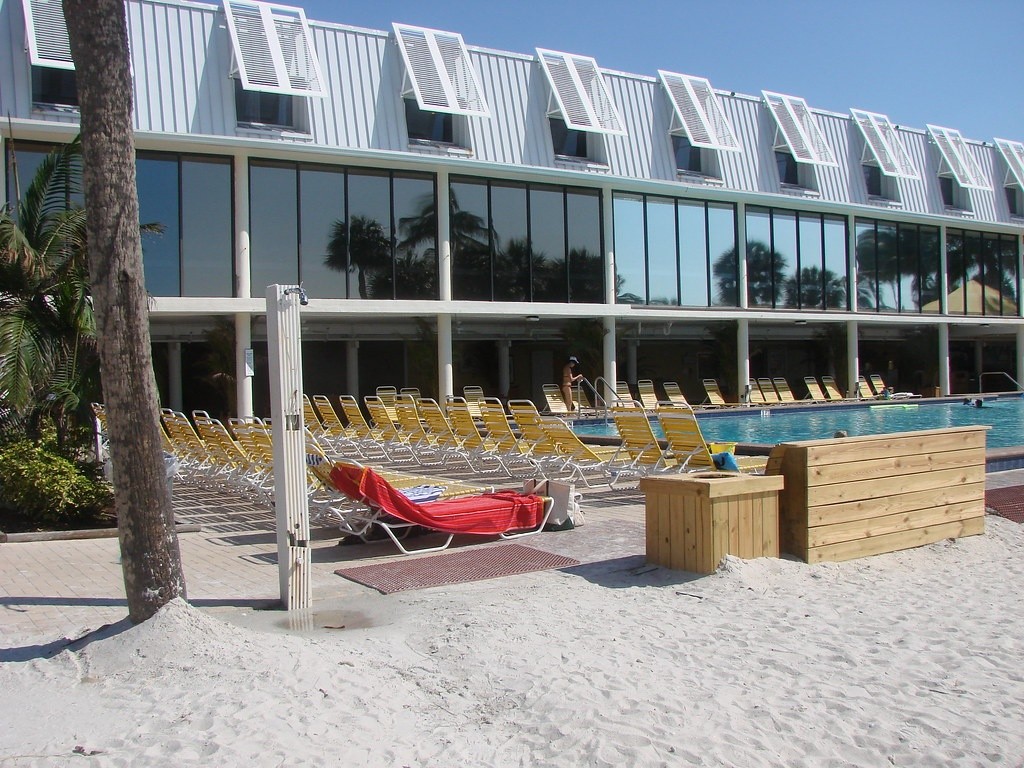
<box><xmin>305</xmin><ymin>452</ymin><xmax>449</xmax><ymax>504</ymax></box>
<box><xmin>327</xmin><ymin>460</ymin><xmax>545</xmax><ymax>535</ymax></box>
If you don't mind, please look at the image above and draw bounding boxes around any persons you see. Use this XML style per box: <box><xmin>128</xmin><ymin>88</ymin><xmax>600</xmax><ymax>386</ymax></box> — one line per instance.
<box><xmin>963</xmin><ymin>398</ymin><xmax>975</xmax><ymax>405</ymax></box>
<box><xmin>562</xmin><ymin>357</ymin><xmax>583</xmax><ymax>416</ymax></box>
<box><xmin>834</xmin><ymin>431</ymin><xmax>847</xmax><ymax>438</ymax></box>
<box><xmin>975</xmin><ymin>399</ymin><xmax>982</xmax><ymax>408</ymax></box>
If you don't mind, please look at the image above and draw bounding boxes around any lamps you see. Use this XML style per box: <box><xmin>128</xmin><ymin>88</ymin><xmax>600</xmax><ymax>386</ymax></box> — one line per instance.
<box><xmin>980</xmin><ymin>324</ymin><xmax>989</xmax><ymax>328</ymax></box>
<box><xmin>795</xmin><ymin>320</ymin><xmax>807</xmax><ymax>324</ymax></box>
<box><xmin>525</xmin><ymin>315</ymin><xmax>539</xmax><ymax>322</ymax></box>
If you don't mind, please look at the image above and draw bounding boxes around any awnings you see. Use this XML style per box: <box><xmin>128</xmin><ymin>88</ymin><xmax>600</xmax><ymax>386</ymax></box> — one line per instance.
<box><xmin>22</xmin><ymin>0</ymin><xmax>1024</xmax><ymax>193</ymax></box>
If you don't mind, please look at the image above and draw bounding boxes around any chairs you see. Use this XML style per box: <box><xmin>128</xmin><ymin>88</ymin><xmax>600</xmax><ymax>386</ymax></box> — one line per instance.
<box><xmin>859</xmin><ymin>375</ymin><xmax>906</xmax><ymax>400</ymax></box>
<box><xmin>870</xmin><ymin>374</ymin><xmax>922</xmax><ymax>398</ymax></box>
<box><xmin>89</xmin><ymin>376</ymin><xmax>858</xmax><ymax>554</ymax></box>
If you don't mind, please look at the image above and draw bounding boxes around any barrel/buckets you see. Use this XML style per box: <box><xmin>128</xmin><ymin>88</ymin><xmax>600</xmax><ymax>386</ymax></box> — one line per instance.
<box><xmin>709</xmin><ymin>442</ymin><xmax>738</xmax><ymax>457</ymax></box>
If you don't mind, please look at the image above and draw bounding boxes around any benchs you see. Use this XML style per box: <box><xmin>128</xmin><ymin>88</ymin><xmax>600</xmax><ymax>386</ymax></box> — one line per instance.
<box><xmin>753</xmin><ymin>420</ymin><xmax>994</xmax><ymax>566</ymax></box>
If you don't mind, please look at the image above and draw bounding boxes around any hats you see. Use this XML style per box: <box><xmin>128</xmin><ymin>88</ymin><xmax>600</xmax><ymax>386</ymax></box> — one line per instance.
<box><xmin>570</xmin><ymin>356</ymin><xmax>579</xmax><ymax>363</ymax></box>
